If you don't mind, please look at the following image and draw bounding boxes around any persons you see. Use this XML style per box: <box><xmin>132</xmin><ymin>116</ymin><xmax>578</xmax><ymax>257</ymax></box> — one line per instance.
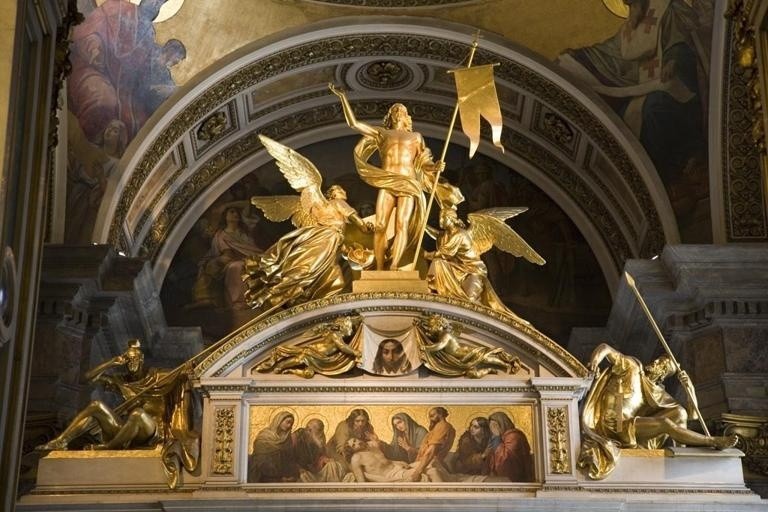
<box><xmin>326</xmin><ymin>408</ymin><xmax>389</xmax><ymax>483</ymax></box>
<box><xmin>325</xmin><ymin>78</ymin><xmax>447</xmax><ymax>268</ymax></box>
<box><xmin>347</xmin><ymin>432</ymin><xmax>510</xmax><ymax>483</ymax></box>
<box><xmin>33</xmin><ymin>337</ymin><xmax>196</xmax><ymax>454</ymax></box>
<box><xmin>244</xmin><ymin>183</ymin><xmax>372</xmax><ymax>308</ymax></box>
<box><xmin>67</xmin><ymin>1</ymin><xmax>157</xmax><ymax>153</ymax></box>
<box><xmin>247</xmin><ymin>412</ymin><xmax>299</xmax><ymax>485</ymax></box>
<box><xmin>356</xmin><ymin>199</ymin><xmax>376</xmax><ymax>219</ymax></box>
<box><xmin>421</xmin><ymin>207</ymin><xmax>528</xmax><ymax>325</ymax></box>
<box><xmin>63</xmin><ymin>117</ymin><xmax>127</xmax><ymax>241</ymax></box>
<box><xmin>204</xmin><ymin>197</ymin><xmax>266</xmax><ymax>312</ymax></box>
<box><xmin>368</xmin><ymin>337</ymin><xmax>412</xmax><ymax>379</ymax></box>
<box><xmin>554</xmin><ymin>0</ymin><xmax>712</xmax><ymax>220</ymax></box>
<box><xmin>289</xmin><ymin>418</ymin><xmax>343</xmax><ymax>485</ymax></box>
<box><xmin>412</xmin><ymin>311</ymin><xmax>521</xmax><ymax>378</ymax></box>
<box><xmin>254</xmin><ymin>315</ymin><xmax>362</xmax><ymax>378</ymax></box>
<box><xmin>584</xmin><ymin>341</ymin><xmax>738</xmax><ymax>453</ymax></box>
<box><xmin>409</xmin><ymin>406</ymin><xmax>455</xmax><ymax>484</ymax></box>
<box><xmin>478</xmin><ymin>410</ymin><xmax>532</xmax><ymax>483</ymax></box>
<box><xmin>442</xmin><ymin>416</ymin><xmax>493</xmax><ymax>475</ymax></box>
<box><xmin>134</xmin><ymin>36</ymin><xmax>188</xmax><ymax>127</ymax></box>
<box><xmin>362</xmin><ymin>412</ymin><xmax>431</xmax><ymax>460</ymax></box>
